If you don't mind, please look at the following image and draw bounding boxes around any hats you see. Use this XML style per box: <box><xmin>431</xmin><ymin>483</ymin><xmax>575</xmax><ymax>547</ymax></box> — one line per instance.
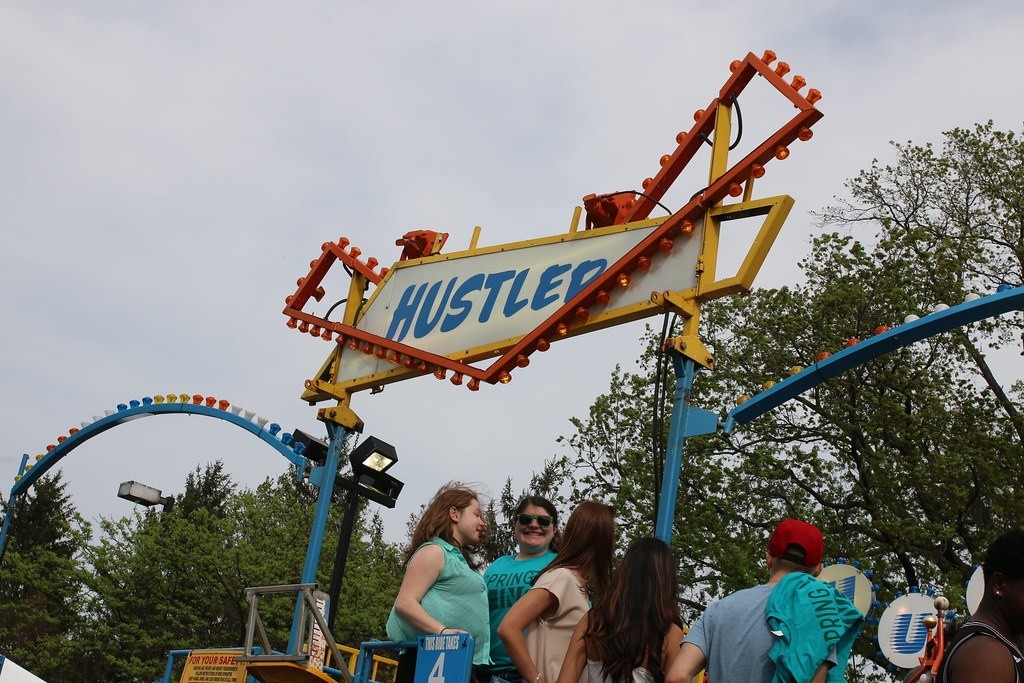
<box><xmin>768</xmin><ymin>519</ymin><xmax>824</xmax><ymax>567</ymax></box>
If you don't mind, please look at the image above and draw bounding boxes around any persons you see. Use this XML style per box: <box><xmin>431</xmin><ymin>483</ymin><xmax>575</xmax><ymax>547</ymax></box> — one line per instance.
<box><xmin>552</xmin><ymin>534</ymin><xmax>686</xmax><ymax>683</ymax></box>
<box><xmin>932</xmin><ymin>522</ymin><xmax>1024</xmax><ymax>683</ymax></box>
<box><xmin>481</xmin><ymin>494</ymin><xmax>565</xmax><ymax>683</ymax></box>
<box><xmin>384</xmin><ymin>488</ymin><xmax>491</xmax><ymax>683</ymax></box>
<box><xmin>496</xmin><ymin>499</ymin><xmax>617</xmax><ymax>683</ymax></box>
<box><xmin>664</xmin><ymin>518</ymin><xmax>839</xmax><ymax>683</ymax></box>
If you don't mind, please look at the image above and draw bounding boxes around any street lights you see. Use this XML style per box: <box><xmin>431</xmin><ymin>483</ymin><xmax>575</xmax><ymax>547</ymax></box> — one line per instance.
<box><xmin>118</xmin><ymin>479</ymin><xmax>175</xmax><ymax>642</ymax></box>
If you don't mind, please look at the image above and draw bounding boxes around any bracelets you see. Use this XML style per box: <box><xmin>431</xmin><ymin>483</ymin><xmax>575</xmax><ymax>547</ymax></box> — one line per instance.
<box><xmin>535</xmin><ymin>671</ymin><xmax>541</xmax><ymax>682</ymax></box>
<box><xmin>440</xmin><ymin>627</ymin><xmax>449</xmax><ymax>635</ymax></box>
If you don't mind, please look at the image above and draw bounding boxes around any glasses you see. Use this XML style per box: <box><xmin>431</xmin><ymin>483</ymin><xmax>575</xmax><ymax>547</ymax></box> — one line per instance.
<box><xmin>516</xmin><ymin>514</ymin><xmax>553</xmax><ymax>527</ymax></box>
